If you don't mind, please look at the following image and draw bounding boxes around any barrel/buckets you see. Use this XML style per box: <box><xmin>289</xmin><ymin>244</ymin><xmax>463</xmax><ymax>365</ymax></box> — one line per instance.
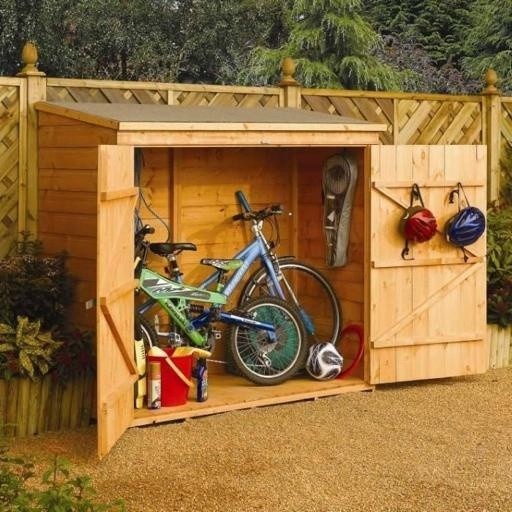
<box><xmin>146</xmin><ymin>347</ymin><xmax>193</xmax><ymax>407</ymax></box>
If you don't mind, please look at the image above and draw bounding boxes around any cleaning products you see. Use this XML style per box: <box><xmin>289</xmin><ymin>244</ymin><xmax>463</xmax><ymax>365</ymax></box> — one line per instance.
<box><xmin>196</xmin><ymin>357</ymin><xmax>209</xmax><ymax>401</ymax></box>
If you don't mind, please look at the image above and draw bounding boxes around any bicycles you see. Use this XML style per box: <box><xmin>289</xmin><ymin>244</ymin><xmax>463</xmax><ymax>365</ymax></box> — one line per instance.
<box><xmin>137</xmin><ymin>206</ymin><xmax>343</xmax><ymax>377</ymax></box>
<box><xmin>134</xmin><ymin>225</ymin><xmax>307</xmax><ymax>386</ymax></box>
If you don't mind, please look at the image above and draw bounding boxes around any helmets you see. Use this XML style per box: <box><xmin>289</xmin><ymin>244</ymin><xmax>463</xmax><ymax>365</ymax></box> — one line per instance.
<box><xmin>444</xmin><ymin>182</ymin><xmax>485</xmax><ymax>263</ymax></box>
<box><xmin>399</xmin><ymin>183</ymin><xmax>437</xmax><ymax>260</ymax></box>
